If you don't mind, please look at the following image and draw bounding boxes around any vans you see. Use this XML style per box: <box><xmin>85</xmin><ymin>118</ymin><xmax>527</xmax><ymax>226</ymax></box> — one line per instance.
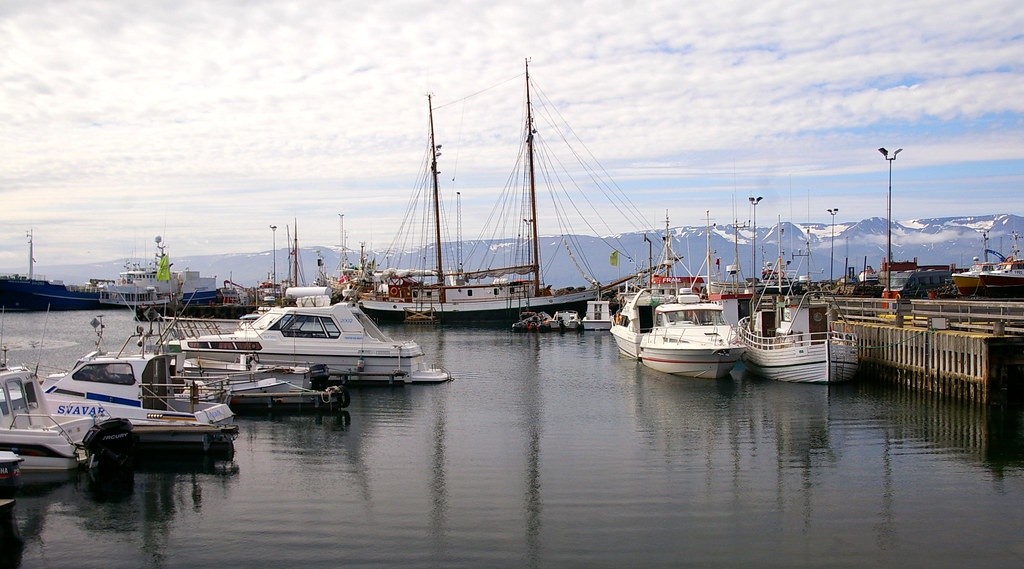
<box><xmin>882</xmin><ymin>271</ymin><xmax>949</xmax><ymax>301</ymax></box>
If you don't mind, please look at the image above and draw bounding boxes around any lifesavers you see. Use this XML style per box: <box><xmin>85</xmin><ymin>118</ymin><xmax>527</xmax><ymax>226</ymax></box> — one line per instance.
<box><xmin>778</xmin><ymin>271</ymin><xmax>784</xmax><ymax>279</ymax></box>
<box><xmin>337</xmin><ymin>385</ymin><xmax>350</xmax><ymax>407</ymax></box>
<box><xmin>390</xmin><ymin>287</ymin><xmax>398</xmax><ymax>295</ymax></box>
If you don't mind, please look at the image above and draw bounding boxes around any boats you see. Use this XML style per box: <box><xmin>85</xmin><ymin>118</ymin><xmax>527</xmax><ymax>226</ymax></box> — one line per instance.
<box><xmin>163</xmin><ymin>283</ymin><xmax>453</xmax><ymax>384</ymax></box>
<box><xmin>549</xmin><ymin>310</ymin><xmax>580</xmax><ymax>330</ymax></box>
<box><xmin>39</xmin><ymin>306</ymin><xmax>239</xmax><ymax>444</ymax></box>
<box><xmin>0</xmin><ymin>227</ymin><xmax>217</xmax><ymax>310</ymax></box>
<box><xmin>512</xmin><ymin>312</ymin><xmax>552</xmax><ymax>333</ymax></box>
<box><xmin>952</xmin><ymin>231</ymin><xmax>1004</xmax><ymax>294</ymax></box>
<box><xmin>706</xmin><ymin>238</ymin><xmax>884</xmax><ymax>298</ymax></box>
<box><xmin>581</xmin><ymin>281</ymin><xmax>612</xmax><ymax>330</ymax></box>
<box><xmin>0</xmin><ymin>450</ymin><xmax>24</xmax><ymax>478</ymax></box>
<box><xmin>737</xmin><ymin>215</ymin><xmax>858</xmax><ymax>385</ymax></box>
<box><xmin>0</xmin><ymin>302</ymin><xmax>112</xmax><ymax>470</ymax></box>
<box><xmin>979</xmin><ymin>232</ymin><xmax>1024</xmax><ymax>296</ymax></box>
<box><xmin>130</xmin><ymin>288</ymin><xmax>346</xmax><ymax>409</ymax></box>
<box><xmin>610</xmin><ymin>207</ymin><xmax>746</xmax><ymax>379</ymax></box>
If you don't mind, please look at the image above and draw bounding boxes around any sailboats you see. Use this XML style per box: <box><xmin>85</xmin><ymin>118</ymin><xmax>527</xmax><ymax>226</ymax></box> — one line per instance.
<box><xmin>343</xmin><ymin>56</ymin><xmax>681</xmax><ymax>325</ymax></box>
<box><xmin>219</xmin><ymin>215</ymin><xmax>369</xmax><ymax>308</ymax></box>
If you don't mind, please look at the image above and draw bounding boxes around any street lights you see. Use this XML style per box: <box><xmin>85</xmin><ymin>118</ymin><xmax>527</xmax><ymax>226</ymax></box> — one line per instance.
<box><xmin>749</xmin><ymin>196</ymin><xmax>763</xmax><ymax>298</ymax></box>
<box><xmin>878</xmin><ymin>148</ymin><xmax>904</xmax><ymax>291</ymax></box>
<box><xmin>826</xmin><ymin>208</ymin><xmax>838</xmax><ymax>291</ymax></box>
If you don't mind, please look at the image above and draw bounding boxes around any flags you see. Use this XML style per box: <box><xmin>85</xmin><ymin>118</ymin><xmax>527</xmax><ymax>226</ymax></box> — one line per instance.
<box><xmin>610</xmin><ymin>251</ymin><xmax>618</xmax><ymax>266</ymax></box>
<box><xmin>157</xmin><ymin>256</ymin><xmax>170</xmax><ymax>281</ymax></box>
